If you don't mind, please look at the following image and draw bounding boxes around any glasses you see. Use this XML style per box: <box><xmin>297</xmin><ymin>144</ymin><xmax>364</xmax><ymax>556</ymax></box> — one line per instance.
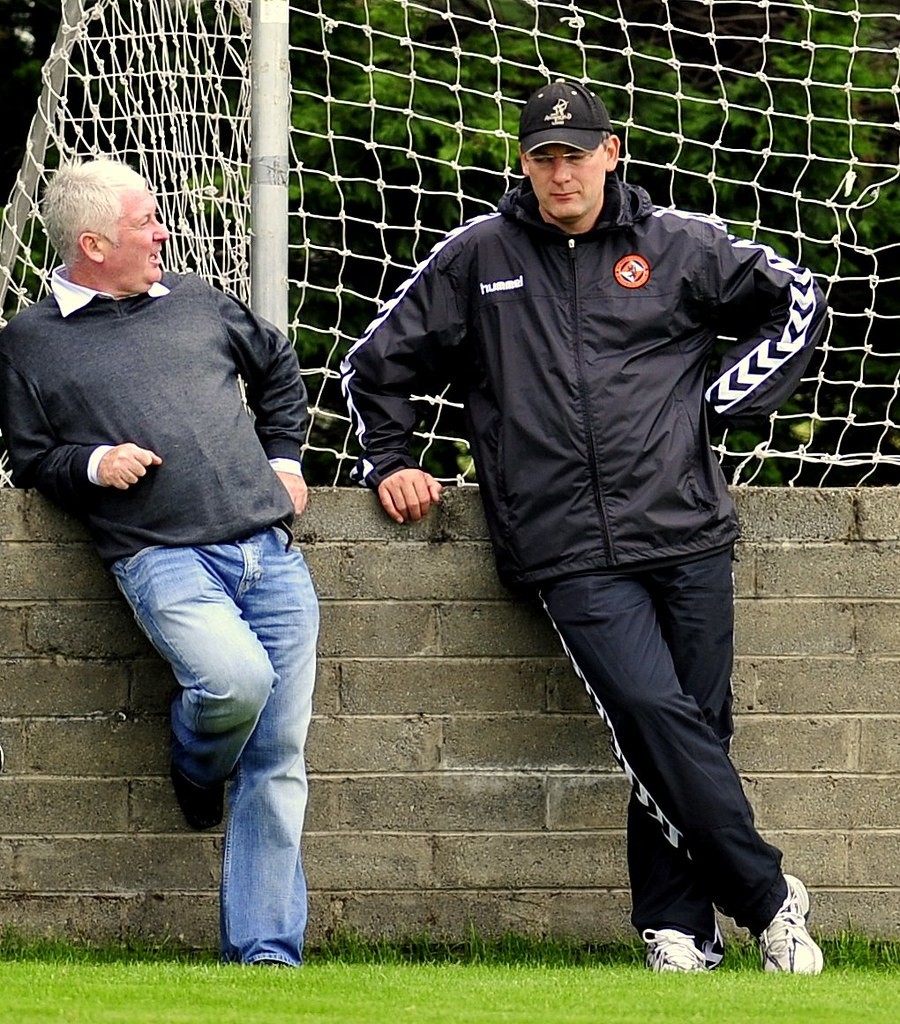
<box><xmin>525</xmin><ymin>150</ymin><xmax>591</xmax><ymax>167</ymax></box>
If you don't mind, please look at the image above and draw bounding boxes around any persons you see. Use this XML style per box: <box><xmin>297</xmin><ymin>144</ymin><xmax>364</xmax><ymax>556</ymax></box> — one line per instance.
<box><xmin>0</xmin><ymin>157</ymin><xmax>321</xmax><ymax>966</ymax></box>
<box><xmin>340</xmin><ymin>81</ymin><xmax>829</xmax><ymax>975</ymax></box>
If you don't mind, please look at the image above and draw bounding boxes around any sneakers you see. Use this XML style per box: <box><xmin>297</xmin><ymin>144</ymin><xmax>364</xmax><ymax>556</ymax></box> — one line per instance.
<box><xmin>642</xmin><ymin>929</ymin><xmax>708</xmax><ymax>973</ymax></box>
<box><xmin>760</xmin><ymin>874</ymin><xmax>823</xmax><ymax>976</ymax></box>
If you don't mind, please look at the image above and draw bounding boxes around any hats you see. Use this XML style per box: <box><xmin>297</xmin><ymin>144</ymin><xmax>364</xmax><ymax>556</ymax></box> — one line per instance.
<box><xmin>517</xmin><ymin>78</ymin><xmax>614</xmax><ymax>153</ymax></box>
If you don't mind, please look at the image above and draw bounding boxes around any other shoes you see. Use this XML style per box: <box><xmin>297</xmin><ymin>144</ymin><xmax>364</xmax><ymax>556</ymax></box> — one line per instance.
<box><xmin>170</xmin><ymin>760</ymin><xmax>225</xmax><ymax>831</ymax></box>
